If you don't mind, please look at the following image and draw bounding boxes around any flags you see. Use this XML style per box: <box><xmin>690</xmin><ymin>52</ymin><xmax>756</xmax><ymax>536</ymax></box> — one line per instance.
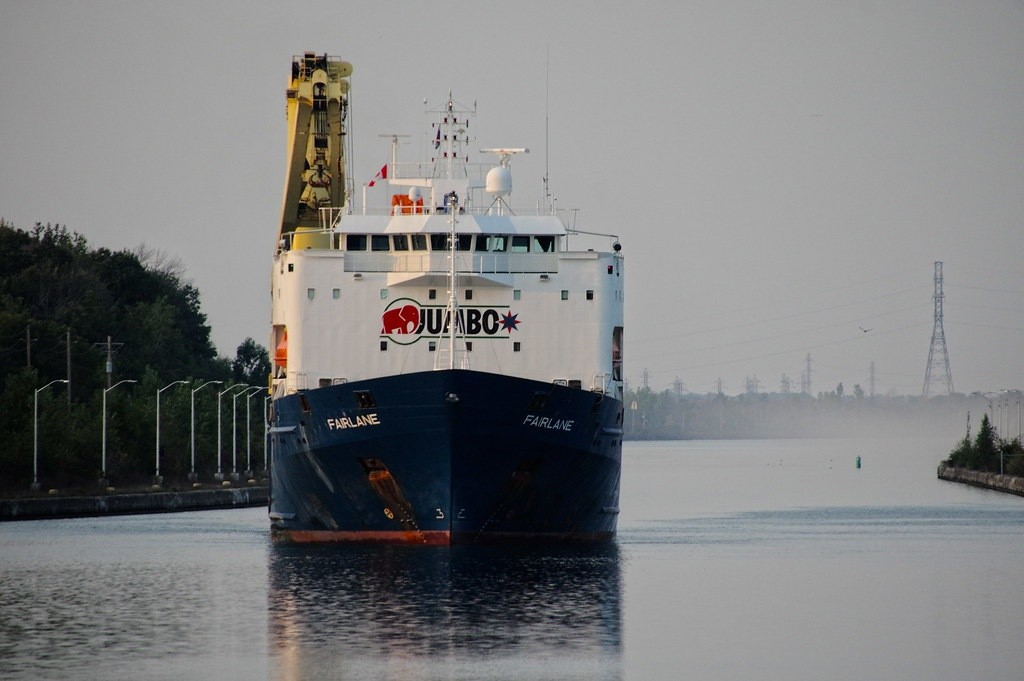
<box><xmin>369</xmin><ymin>164</ymin><xmax>386</xmax><ymax>186</ymax></box>
<box><xmin>435</xmin><ymin>125</ymin><xmax>440</xmax><ymax>149</ymax></box>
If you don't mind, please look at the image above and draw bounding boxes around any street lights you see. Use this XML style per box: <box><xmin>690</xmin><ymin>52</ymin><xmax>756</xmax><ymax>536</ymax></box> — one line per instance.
<box><xmin>247</xmin><ymin>387</ymin><xmax>269</xmax><ymax>471</ymax></box>
<box><xmin>34</xmin><ymin>379</ymin><xmax>69</xmax><ymax>483</ymax></box>
<box><xmin>102</xmin><ymin>380</ymin><xmax>138</xmax><ymax>477</ymax></box>
<box><xmin>233</xmin><ymin>386</ymin><xmax>263</xmax><ymax>473</ymax></box>
<box><xmin>218</xmin><ymin>384</ymin><xmax>249</xmax><ymax>473</ymax></box>
<box><xmin>191</xmin><ymin>381</ymin><xmax>223</xmax><ymax>472</ymax></box>
<box><xmin>156</xmin><ymin>380</ymin><xmax>189</xmax><ymax>475</ymax></box>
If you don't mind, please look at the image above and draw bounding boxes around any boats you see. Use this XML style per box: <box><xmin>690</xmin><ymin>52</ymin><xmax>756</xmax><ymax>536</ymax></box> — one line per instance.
<box><xmin>266</xmin><ymin>50</ymin><xmax>626</xmax><ymax>549</ymax></box>
